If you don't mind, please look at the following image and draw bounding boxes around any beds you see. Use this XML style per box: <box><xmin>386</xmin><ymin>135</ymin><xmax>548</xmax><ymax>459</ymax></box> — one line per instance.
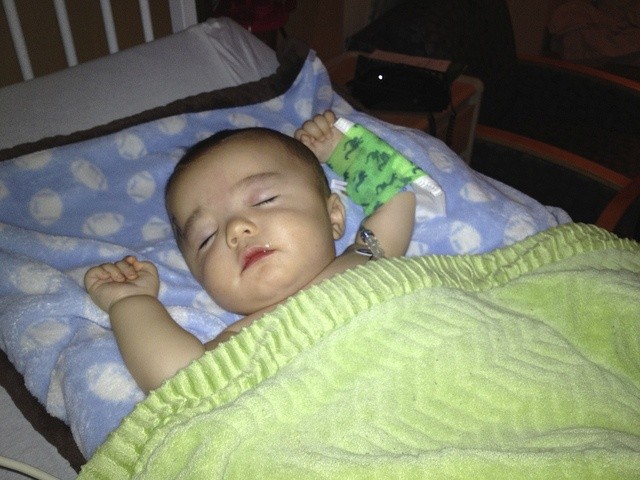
<box><xmin>1</xmin><ymin>1</ymin><xmax>636</xmax><ymax>479</ymax></box>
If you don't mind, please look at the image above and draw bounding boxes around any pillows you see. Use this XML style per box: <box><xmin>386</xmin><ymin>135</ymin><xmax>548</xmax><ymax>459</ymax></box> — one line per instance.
<box><xmin>1</xmin><ymin>47</ymin><xmax>574</xmax><ymax>465</ymax></box>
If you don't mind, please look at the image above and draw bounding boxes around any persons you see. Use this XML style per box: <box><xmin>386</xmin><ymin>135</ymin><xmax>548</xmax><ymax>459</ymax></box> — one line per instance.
<box><xmin>85</xmin><ymin>109</ymin><xmax>416</xmax><ymax>400</ymax></box>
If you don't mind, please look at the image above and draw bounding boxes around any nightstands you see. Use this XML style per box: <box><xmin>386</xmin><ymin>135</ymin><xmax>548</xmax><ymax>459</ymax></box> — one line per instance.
<box><xmin>323</xmin><ymin>48</ymin><xmax>485</xmax><ymax>166</ymax></box>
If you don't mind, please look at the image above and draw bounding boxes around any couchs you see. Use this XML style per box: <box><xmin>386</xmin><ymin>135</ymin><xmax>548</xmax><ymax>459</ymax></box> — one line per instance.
<box><xmin>345</xmin><ymin>0</ymin><xmax>635</xmax><ymax>232</ymax></box>
